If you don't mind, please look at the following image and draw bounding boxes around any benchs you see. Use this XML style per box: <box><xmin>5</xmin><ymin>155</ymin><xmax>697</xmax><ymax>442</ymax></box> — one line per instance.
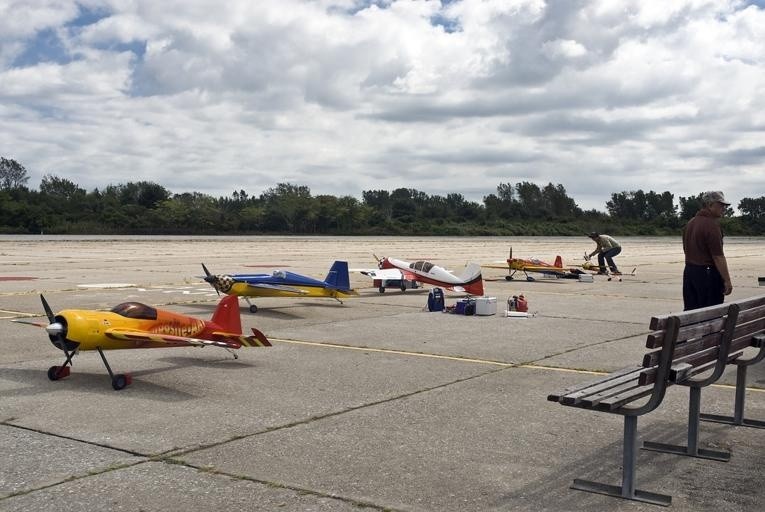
<box><xmin>547</xmin><ymin>296</ymin><xmax>764</xmax><ymax>507</ymax></box>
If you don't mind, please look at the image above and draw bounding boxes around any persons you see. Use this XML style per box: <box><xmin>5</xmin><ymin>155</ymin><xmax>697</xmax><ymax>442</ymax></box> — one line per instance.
<box><xmin>683</xmin><ymin>192</ymin><xmax>732</xmax><ymax>309</ymax></box>
<box><xmin>584</xmin><ymin>232</ymin><xmax>621</xmax><ymax>275</ymax></box>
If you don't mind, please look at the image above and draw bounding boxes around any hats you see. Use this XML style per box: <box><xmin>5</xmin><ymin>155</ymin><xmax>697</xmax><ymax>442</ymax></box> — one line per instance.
<box><xmin>588</xmin><ymin>232</ymin><xmax>599</xmax><ymax>238</ymax></box>
<box><xmin>702</xmin><ymin>191</ymin><xmax>730</xmax><ymax>206</ymax></box>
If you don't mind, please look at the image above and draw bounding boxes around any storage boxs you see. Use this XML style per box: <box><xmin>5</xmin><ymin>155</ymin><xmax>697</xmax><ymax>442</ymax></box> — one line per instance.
<box><xmin>475</xmin><ymin>297</ymin><xmax>497</xmax><ymax>315</ymax></box>
